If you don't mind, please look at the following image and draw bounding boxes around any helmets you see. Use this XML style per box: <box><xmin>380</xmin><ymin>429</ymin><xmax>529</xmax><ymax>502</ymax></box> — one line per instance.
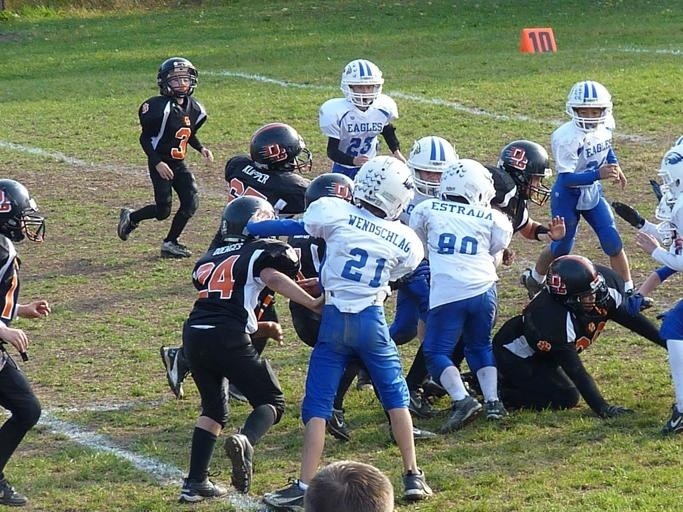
<box><xmin>565</xmin><ymin>80</ymin><xmax>613</xmax><ymax>134</ymax></box>
<box><xmin>219</xmin><ymin>195</ymin><xmax>277</xmax><ymax>238</ymax></box>
<box><xmin>544</xmin><ymin>254</ymin><xmax>611</xmax><ymax>318</ymax></box>
<box><xmin>439</xmin><ymin>158</ymin><xmax>498</xmax><ymax>205</ymax></box>
<box><xmin>496</xmin><ymin>139</ymin><xmax>553</xmax><ymax>207</ymax></box>
<box><xmin>352</xmin><ymin>155</ymin><xmax>415</xmax><ymax>221</ymax></box>
<box><xmin>0</xmin><ymin>179</ymin><xmax>47</xmax><ymax>244</ymax></box>
<box><xmin>249</xmin><ymin>122</ymin><xmax>313</xmax><ymax>176</ymax></box>
<box><xmin>653</xmin><ymin>135</ymin><xmax>683</xmax><ymax>250</ymax></box>
<box><xmin>303</xmin><ymin>172</ymin><xmax>354</xmax><ymax>210</ymax></box>
<box><xmin>407</xmin><ymin>136</ymin><xmax>460</xmax><ymax>199</ymax></box>
<box><xmin>156</xmin><ymin>56</ymin><xmax>200</xmax><ymax>100</ymax></box>
<box><xmin>339</xmin><ymin>58</ymin><xmax>385</xmax><ymax>108</ymax></box>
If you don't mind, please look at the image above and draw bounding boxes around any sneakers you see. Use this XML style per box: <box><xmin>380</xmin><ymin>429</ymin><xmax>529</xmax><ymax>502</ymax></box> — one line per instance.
<box><xmin>117</xmin><ymin>207</ymin><xmax>140</xmax><ymax>241</ymax></box>
<box><xmin>0</xmin><ymin>478</ymin><xmax>29</xmax><ymax>506</ymax></box>
<box><xmin>519</xmin><ymin>268</ymin><xmax>543</xmax><ymax>300</ymax></box>
<box><xmin>387</xmin><ymin>424</ymin><xmax>436</xmax><ymax>443</ymax></box>
<box><xmin>624</xmin><ymin>288</ymin><xmax>654</xmax><ymax>311</ymax></box>
<box><xmin>356</xmin><ymin>377</ymin><xmax>374</xmax><ymax>390</ymax></box>
<box><xmin>401</xmin><ymin>469</ymin><xmax>434</xmax><ymax>500</ymax></box>
<box><xmin>482</xmin><ymin>396</ymin><xmax>511</xmax><ymax>420</ymax></box>
<box><xmin>421</xmin><ymin>372</ymin><xmax>484</xmax><ymax>396</ymax></box>
<box><xmin>178</xmin><ymin>474</ymin><xmax>228</xmax><ymax>502</ymax></box>
<box><xmin>325</xmin><ymin>407</ymin><xmax>353</xmax><ymax>441</ymax></box>
<box><xmin>408</xmin><ymin>391</ymin><xmax>433</xmax><ymax>419</ymax></box>
<box><xmin>439</xmin><ymin>394</ymin><xmax>485</xmax><ymax>434</ymax></box>
<box><xmin>229</xmin><ymin>383</ymin><xmax>248</xmax><ymax>402</ymax></box>
<box><xmin>160</xmin><ymin>345</ymin><xmax>192</xmax><ymax>400</ymax></box>
<box><xmin>160</xmin><ymin>238</ymin><xmax>192</xmax><ymax>258</ymax></box>
<box><xmin>223</xmin><ymin>427</ymin><xmax>256</xmax><ymax>493</ymax></box>
<box><xmin>262</xmin><ymin>479</ymin><xmax>306</xmax><ymax>512</ymax></box>
<box><xmin>661</xmin><ymin>403</ymin><xmax>683</xmax><ymax>436</ymax></box>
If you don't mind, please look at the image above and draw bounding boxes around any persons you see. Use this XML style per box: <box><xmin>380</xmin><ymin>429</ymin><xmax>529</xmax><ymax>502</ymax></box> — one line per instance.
<box><xmin>115</xmin><ymin>55</ymin><xmax>215</xmax><ymax>261</ymax></box>
<box><xmin>517</xmin><ymin>80</ymin><xmax>654</xmax><ymax>314</ymax></box>
<box><xmin>316</xmin><ymin>57</ymin><xmax>410</xmax><ymax>179</ymax></box>
<box><xmin>159</xmin><ymin>123</ymin><xmax>683</xmax><ymax>512</ymax></box>
<box><xmin>0</xmin><ymin>175</ymin><xmax>51</xmax><ymax>507</ymax></box>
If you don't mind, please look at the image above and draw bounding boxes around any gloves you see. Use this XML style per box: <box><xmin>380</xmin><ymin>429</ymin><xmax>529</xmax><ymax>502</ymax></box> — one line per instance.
<box><xmin>649</xmin><ymin>178</ymin><xmax>663</xmax><ymax>202</ymax></box>
<box><xmin>610</xmin><ymin>201</ymin><xmax>645</xmax><ymax>229</ymax></box>
<box><xmin>602</xmin><ymin>404</ymin><xmax>635</xmax><ymax>419</ymax></box>
<box><xmin>396</xmin><ymin>260</ymin><xmax>431</xmax><ymax>287</ymax></box>
